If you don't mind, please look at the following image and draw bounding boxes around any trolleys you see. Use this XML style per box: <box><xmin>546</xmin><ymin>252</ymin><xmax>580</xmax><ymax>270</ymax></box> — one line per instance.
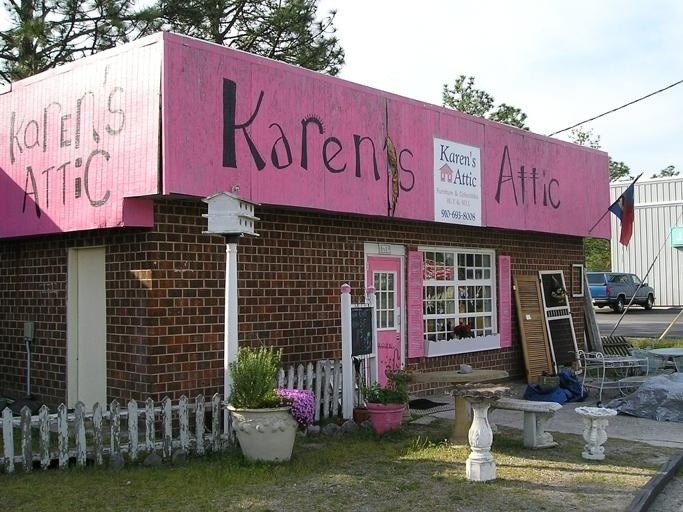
<box><xmin>569</xmin><ymin>347</ymin><xmax>650</xmax><ymax>410</ymax></box>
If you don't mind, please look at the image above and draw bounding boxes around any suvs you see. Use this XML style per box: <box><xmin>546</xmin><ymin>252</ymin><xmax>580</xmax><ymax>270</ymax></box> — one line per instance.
<box><xmin>586</xmin><ymin>271</ymin><xmax>657</xmax><ymax>313</ymax></box>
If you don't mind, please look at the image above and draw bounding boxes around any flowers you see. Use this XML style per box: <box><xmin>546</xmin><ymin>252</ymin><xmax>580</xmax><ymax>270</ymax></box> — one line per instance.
<box><xmin>224</xmin><ymin>345</ymin><xmax>317</xmax><ymax>433</ymax></box>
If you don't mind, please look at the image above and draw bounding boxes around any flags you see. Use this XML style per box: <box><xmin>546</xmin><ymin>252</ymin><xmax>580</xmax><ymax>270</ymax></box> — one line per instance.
<box><xmin>608</xmin><ymin>182</ymin><xmax>636</xmax><ymax>248</ymax></box>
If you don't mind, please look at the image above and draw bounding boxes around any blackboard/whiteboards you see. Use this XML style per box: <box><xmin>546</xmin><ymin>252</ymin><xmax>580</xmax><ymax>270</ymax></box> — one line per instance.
<box><xmin>351</xmin><ymin>304</ymin><xmax>376</xmax><ymax>361</ymax></box>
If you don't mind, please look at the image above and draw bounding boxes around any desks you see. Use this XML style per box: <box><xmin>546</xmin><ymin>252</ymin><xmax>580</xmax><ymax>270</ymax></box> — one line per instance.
<box><xmin>418</xmin><ymin>367</ymin><xmax>510</xmax><ymax>447</ymax></box>
<box><xmin>441</xmin><ymin>381</ymin><xmax>521</xmax><ymax>485</ymax></box>
<box><xmin>649</xmin><ymin>347</ymin><xmax>682</xmax><ymax>373</ymax></box>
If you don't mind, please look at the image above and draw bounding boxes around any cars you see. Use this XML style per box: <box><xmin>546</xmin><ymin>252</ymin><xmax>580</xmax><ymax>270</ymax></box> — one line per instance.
<box><xmin>424</xmin><ymin>277</ymin><xmax>492</xmax><ymax>314</ymax></box>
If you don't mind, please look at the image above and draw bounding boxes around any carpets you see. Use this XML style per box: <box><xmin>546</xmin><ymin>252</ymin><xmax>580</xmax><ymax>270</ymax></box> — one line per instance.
<box><xmin>408</xmin><ymin>397</ymin><xmax>449</xmax><ymax>411</ymax></box>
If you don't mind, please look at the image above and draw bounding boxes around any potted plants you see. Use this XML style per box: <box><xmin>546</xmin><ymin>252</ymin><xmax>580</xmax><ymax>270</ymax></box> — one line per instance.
<box><xmin>357</xmin><ymin>366</ymin><xmax>411</xmax><ymax>435</ymax></box>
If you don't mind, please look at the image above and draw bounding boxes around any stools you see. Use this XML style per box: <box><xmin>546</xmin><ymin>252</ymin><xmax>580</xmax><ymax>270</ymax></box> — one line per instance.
<box><xmin>573</xmin><ymin>404</ymin><xmax>618</xmax><ymax>460</ymax></box>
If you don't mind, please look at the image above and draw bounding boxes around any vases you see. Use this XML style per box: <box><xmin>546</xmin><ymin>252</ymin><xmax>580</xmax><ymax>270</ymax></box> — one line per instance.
<box><xmin>224</xmin><ymin>402</ymin><xmax>299</xmax><ymax>461</ymax></box>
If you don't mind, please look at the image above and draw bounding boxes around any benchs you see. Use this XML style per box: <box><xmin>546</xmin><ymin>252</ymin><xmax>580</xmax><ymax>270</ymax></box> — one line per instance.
<box><xmin>487</xmin><ymin>395</ymin><xmax>562</xmax><ymax>450</ymax></box>
